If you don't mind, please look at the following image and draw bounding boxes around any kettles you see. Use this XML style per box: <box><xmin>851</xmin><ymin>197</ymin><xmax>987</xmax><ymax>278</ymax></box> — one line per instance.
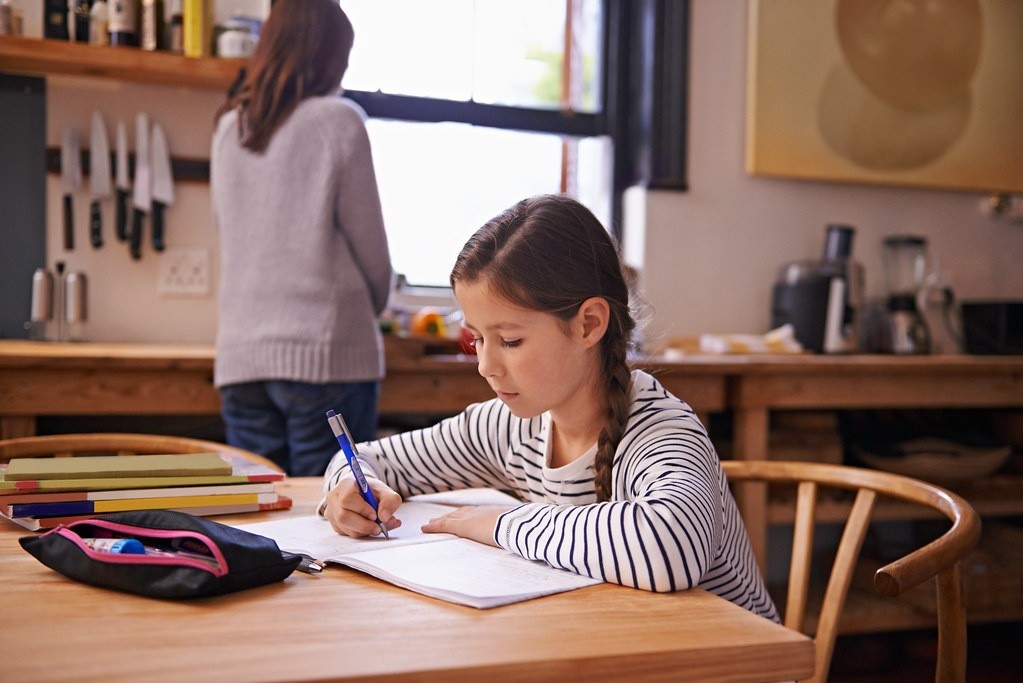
<box><xmin>769</xmin><ymin>219</ymin><xmax>860</xmax><ymax>355</ymax></box>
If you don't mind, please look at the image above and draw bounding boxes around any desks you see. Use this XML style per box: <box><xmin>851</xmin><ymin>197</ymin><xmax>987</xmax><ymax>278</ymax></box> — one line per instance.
<box><xmin>0</xmin><ymin>465</ymin><xmax>821</xmax><ymax>683</ymax></box>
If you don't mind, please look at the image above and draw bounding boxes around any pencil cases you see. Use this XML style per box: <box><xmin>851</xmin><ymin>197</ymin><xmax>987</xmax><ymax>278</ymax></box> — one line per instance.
<box><xmin>18</xmin><ymin>509</ymin><xmax>302</xmax><ymax>600</ymax></box>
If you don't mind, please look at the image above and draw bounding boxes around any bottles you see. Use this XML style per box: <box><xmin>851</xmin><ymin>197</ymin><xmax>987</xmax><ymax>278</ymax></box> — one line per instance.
<box><xmin>38</xmin><ymin>1</ymin><xmax>215</xmax><ymax>60</ymax></box>
<box><xmin>217</xmin><ymin>27</ymin><xmax>259</xmax><ymax>58</ymax></box>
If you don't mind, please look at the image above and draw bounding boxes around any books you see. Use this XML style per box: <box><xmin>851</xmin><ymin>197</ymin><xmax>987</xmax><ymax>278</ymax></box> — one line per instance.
<box><xmin>12</xmin><ymin>494</ymin><xmax>293</xmax><ymax>531</ymax></box>
<box><xmin>0</xmin><ymin>456</ymin><xmax>285</xmax><ymax>497</ymax></box>
<box><xmin>229</xmin><ymin>497</ymin><xmax>607</xmax><ymax>612</ymax></box>
<box><xmin>0</xmin><ymin>489</ymin><xmax>279</xmax><ymax>519</ymax></box>
<box><xmin>0</xmin><ymin>482</ymin><xmax>275</xmax><ymax>504</ymax></box>
<box><xmin>5</xmin><ymin>453</ymin><xmax>231</xmax><ymax>481</ymax></box>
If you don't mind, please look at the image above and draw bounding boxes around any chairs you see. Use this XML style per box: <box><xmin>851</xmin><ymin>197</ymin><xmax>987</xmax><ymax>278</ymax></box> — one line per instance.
<box><xmin>0</xmin><ymin>432</ymin><xmax>286</xmax><ymax>474</ymax></box>
<box><xmin>716</xmin><ymin>459</ymin><xmax>984</xmax><ymax>683</ymax></box>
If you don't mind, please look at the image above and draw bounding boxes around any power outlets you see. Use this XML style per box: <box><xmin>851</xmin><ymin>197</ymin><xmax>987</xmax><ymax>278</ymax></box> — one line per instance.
<box><xmin>161</xmin><ymin>247</ymin><xmax>208</xmax><ymax>297</ymax></box>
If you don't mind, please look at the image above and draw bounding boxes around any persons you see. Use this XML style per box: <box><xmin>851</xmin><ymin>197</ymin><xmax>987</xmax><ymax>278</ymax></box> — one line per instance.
<box><xmin>313</xmin><ymin>192</ymin><xmax>789</xmax><ymax>626</ymax></box>
<box><xmin>207</xmin><ymin>2</ymin><xmax>394</xmax><ymax>478</ymax></box>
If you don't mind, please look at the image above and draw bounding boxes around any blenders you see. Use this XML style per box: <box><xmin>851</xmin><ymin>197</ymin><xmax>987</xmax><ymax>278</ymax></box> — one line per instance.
<box><xmin>866</xmin><ymin>231</ymin><xmax>933</xmax><ymax>354</ymax></box>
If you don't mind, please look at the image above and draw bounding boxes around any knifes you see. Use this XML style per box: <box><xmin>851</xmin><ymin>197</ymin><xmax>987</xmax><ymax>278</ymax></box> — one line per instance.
<box><xmin>59</xmin><ymin>120</ymin><xmax>83</xmax><ymax>250</ymax></box>
<box><xmin>114</xmin><ymin>117</ymin><xmax>130</xmax><ymax>240</ymax></box>
<box><xmin>150</xmin><ymin>121</ymin><xmax>174</xmax><ymax>252</ymax></box>
<box><xmin>87</xmin><ymin>108</ymin><xmax>112</xmax><ymax>249</ymax></box>
<box><xmin>129</xmin><ymin>108</ymin><xmax>151</xmax><ymax>260</ymax></box>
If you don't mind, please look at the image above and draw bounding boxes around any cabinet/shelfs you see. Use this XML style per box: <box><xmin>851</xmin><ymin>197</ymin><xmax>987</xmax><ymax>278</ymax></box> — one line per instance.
<box><xmin>0</xmin><ymin>339</ymin><xmax>220</xmax><ymax>439</ymax></box>
<box><xmin>374</xmin><ymin>339</ymin><xmax>1023</xmax><ymax>634</ymax></box>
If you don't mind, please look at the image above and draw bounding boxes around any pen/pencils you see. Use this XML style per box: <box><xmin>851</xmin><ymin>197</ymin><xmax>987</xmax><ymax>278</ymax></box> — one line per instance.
<box><xmin>281</xmin><ymin>551</ymin><xmax>328</xmax><ymax>574</ymax></box>
<box><xmin>327</xmin><ymin>409</ymin><xmax>390</xmax><ymax>540</ymax></box>
